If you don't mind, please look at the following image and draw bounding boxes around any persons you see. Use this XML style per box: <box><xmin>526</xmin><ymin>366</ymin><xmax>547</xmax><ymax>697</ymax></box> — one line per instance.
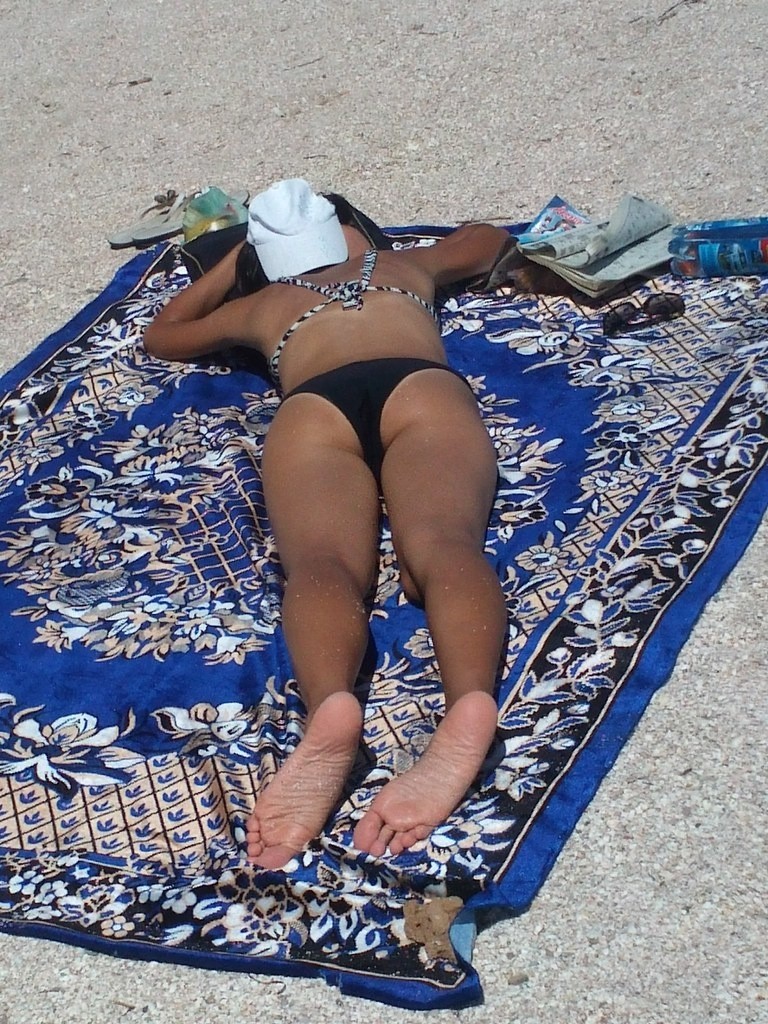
<box><xmin>142</xmin><ymin>177</ymin><xmax>511</xmax><ymax>869</ymax></box>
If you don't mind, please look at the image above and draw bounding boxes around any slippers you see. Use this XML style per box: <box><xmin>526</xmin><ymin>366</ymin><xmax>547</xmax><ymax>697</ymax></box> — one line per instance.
<box><xmin>130</xmin><ymin>186</ymin><xmax>215</xmax><ymax>249</ymax></box>
<box><xmin>108</xmin><ymin>189</ymin><xmax>194</xmax><ymax>249</ymax></box>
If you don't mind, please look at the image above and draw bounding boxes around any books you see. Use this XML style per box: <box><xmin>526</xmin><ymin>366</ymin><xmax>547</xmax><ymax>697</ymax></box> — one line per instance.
<box><xmin>481</xmin><ymin>192</ymin><xmax>684</xmax><ymax>299</ymax></box>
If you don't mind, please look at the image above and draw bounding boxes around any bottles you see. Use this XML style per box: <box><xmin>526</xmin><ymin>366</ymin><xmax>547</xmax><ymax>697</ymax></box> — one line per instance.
<box><xmin>666</xmin><ymin>216</ymin><xmax>768</xmax><ymax>278</ymax></box>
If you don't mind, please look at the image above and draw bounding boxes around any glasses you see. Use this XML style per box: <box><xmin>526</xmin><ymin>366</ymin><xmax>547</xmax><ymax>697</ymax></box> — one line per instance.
<box><xmin>603</xmin><ymin>293</ymin><xmax>685</xmax><ymax>336</ymax></box>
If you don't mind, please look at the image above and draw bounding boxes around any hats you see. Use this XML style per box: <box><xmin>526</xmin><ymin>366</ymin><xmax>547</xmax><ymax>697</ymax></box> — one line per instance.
<box><xmin>246</xmin><ymin>179</ymin><xmax>348</xmax><ymax>281</ymax></box>
